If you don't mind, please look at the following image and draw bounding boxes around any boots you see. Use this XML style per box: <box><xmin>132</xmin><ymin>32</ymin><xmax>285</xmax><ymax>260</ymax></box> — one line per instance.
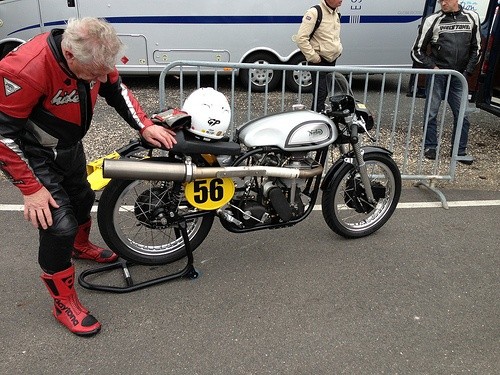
<box><xmin>71</xmin><ymin>216</ymin><xmax>119</xmax><ymax>262</ymax></box>
<box><xmin>38</xmin><ymin>264</ymin><xmax>102</xmax><ymax>337</ymax></box>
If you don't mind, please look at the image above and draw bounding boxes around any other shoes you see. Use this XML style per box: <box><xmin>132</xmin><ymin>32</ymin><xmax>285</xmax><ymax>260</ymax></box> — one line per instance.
<box><xmin>450</xmin><ymin>146</ymin><xmax>467</xmax><ymax>156</ymax></box>
<box><xmin>424</xmin><ymin>148</ymin><xmax>436</xmax><ymax>160</ymax></box>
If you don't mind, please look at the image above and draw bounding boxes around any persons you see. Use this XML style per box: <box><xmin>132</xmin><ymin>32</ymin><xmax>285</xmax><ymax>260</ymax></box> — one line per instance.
<box><xmin>296</xmin><ymin>0</ymin><xmax>344</xmax><ymax>113</ymax></box>
<box><xmin>0</xmin><ymin>17</ymin><xmax>177</xmax><ymax>337</ymax></box>
<box><xmin>411</xmin><ymin>0</ymin><xmax>483</xmax><ymax>165</ymax></box>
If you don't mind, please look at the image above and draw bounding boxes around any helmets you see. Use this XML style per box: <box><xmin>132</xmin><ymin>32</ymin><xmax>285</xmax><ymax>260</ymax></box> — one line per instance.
<box><xmin>180</xmin><ymin>87</ymin><xmax>232</xmax><ymax>142</ymax></box>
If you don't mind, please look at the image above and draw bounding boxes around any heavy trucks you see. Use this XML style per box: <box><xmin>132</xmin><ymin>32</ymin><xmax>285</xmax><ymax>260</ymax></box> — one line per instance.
<box><xmin>0</xmin><ymin>0</ymin><xmax>498</xmax><ymax>103</ymax></box>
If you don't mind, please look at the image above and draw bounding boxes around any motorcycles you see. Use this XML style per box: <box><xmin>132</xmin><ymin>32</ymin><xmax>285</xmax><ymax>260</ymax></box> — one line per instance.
<box><xmin>95</xmin><ymin>71</ymin><xmax>403</xmax><ymax>266</ymax></box>
